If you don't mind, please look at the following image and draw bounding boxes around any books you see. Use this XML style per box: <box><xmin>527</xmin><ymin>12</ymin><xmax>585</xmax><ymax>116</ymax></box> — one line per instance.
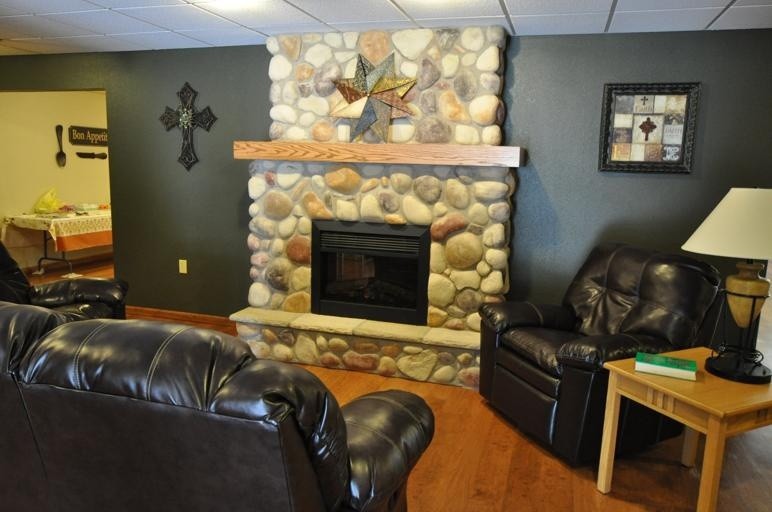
<box><xmin>634</xmin><ymin>351</ymin><xmax>698</xmax><ymax>383</ymax></box>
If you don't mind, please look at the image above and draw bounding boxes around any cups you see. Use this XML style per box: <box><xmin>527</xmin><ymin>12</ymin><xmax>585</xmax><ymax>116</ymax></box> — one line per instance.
<box><xmin>337</xmin><ymin>124</ymin><xmax>351</xmax><ymax>142</ymax></box>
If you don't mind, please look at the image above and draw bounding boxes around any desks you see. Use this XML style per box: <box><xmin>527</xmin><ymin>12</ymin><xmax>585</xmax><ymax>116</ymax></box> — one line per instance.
<box><xmin>1</xmin><ymin>204</ymin><xmax>115</xmax><ymax>281</ymax></box>
<box><xmin>590</xmin><ymin>344</ymin><xmax>772</xmax><ymax>511</ymax></box>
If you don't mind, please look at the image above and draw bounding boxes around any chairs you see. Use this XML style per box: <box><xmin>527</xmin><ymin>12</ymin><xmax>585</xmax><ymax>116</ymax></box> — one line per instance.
<box><xmin>1</xmin><ymin>237</ymin><xmax>134</xmax><ymax>325</ymax></box>
<box><xmin>472</xmin><ymin>235</ymin><xmax>726</xmax><ymax>473</ymax></box>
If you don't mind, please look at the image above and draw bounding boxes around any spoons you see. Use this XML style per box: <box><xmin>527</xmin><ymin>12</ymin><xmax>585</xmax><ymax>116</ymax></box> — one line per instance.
<box><xmin>55</xmin><ymin>125</ymin><xmax>67</xmax><ymax>167</ymax></box>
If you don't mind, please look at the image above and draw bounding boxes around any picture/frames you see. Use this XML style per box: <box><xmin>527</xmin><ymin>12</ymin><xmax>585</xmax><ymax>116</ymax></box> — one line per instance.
<box><xmin>596</xmin><ymin>80</ymin><xmax>701</xmax><ymax>175</ymax></box>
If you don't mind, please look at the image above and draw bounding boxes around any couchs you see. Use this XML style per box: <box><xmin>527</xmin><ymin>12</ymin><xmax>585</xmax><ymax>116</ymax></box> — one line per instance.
<box><xmin>1</xmin><ymin>295</ymin><xmax>437</xmax><ymax>511</ymax></box>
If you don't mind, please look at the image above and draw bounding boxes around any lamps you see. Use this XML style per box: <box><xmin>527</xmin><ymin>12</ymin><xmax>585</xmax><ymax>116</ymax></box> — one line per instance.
<box><xmin>680</xmin><ymin>187</ymin><xmax>771</xmax><ymax>387</ymax></box>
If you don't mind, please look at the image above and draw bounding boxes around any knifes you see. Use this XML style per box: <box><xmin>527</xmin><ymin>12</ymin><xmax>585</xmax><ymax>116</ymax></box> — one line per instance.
<box><xmin>76</xmin><ymin>151</ymin><xmax>107</xmax><ymax>159</ymax></box>
